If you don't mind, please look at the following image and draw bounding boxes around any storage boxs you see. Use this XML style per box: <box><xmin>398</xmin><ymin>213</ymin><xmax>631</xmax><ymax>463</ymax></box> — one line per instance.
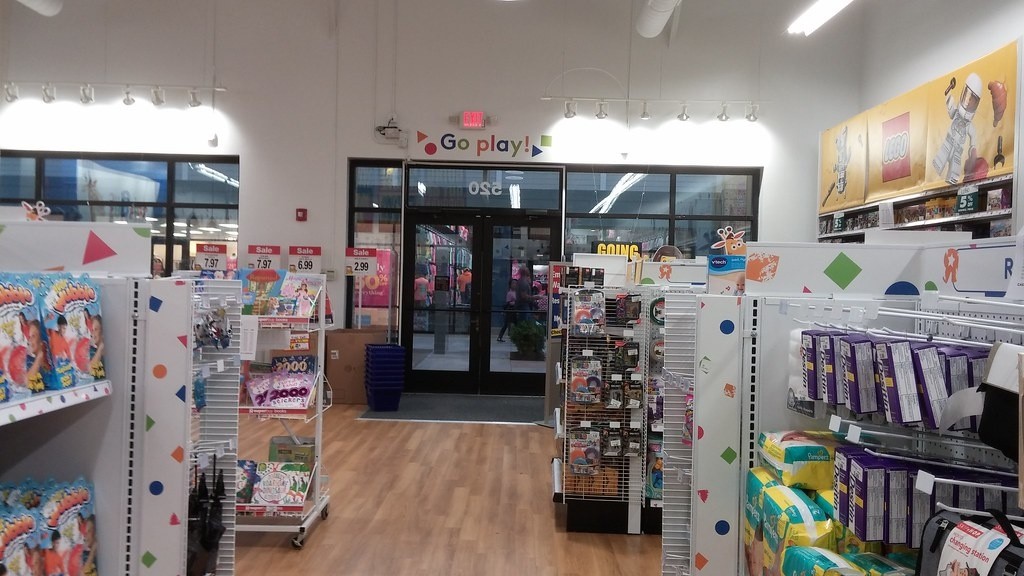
<box><xmin>801</xmin><ymin>329</ymin><xmax>1024</xmax><ymax>545</ymax></box>
<box><xmin>363</xmin><ymin>343</ymin><xmax>405</xmax><ymax>411</ymax></box>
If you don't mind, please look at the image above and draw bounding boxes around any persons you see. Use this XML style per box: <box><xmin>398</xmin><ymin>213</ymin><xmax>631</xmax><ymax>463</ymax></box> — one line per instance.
<box><xmin>19</xmin><ymin>308</ymin><xmax>106</xmax><ymax>397</ymax></box>
<box><xmin>414</xmin><ymin>271</ymin><xmax>430</xmax><ymax>308</ymax></box>
<box><xmin>456</xmin><ymin>268</ymin><xmax>472</xmax><ymax>303</ymax></box>
<box><xmin>496</xmin><ymin>279</ymin><xmax>517</xmax><ymax>342</ymax></box>
<box><xmin>0</xmin><ymin>512</ymin><xmax>97</xmax><ymax>576</ymax></box>
<box><xmin>747</xmin><ymin>539</ymin><xmax>772</xmax><ymax>576</ymax></box>
<box><xmin>515</xmin><ymin>265</ymin><xmax>544</xmax><ymax>321</ymax></box>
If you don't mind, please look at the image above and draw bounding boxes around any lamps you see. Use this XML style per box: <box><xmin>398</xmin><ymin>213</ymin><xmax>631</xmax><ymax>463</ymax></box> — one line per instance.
<box><xmin>42</xmin><ymin>83</ymin><xmax>56</xmax><ymax>104</ymax></box>
<box><xmin>151</xmin><ymin>86</ymin><xmax>165</xmax><ymax>106</ymax></box>
<box><xmin>640</xmin><ymin>103</ymin><xmax>651</xmax><ymax>119</ymax></box>
<box><xmin>79</xmin><ymin>83</ymin><xmax>95</xmax><ymax>105</ymax></box>
<box><xmin>187</xmin><ymin>89</ymin><xmax>202</xmax><ymax>108</ymax></box>
<box><xmin>122</xmin><ymin>84</ymin><xmax>135</xmax><ymax>105</ymax></box>
<box><xmin>4</xmin><ymin>82</ymin><xmax>19</xmax><ymax>102</ymax></box>
<box><xmin>717</xmin><ymin>106</ymin><xmax>730</xmax><ymax>121</ymax></box>
<box><xmin>781</xmin><ymin>0</ymin><xmax>853</xmax><ymax>37</ymax></box>
<box><xmin>509</xmin><ymin>184</ymin><xmax>521</xmax><ymax>208</ymax></box>
<box><xmin>564</xmin><ymin>100</ymin><xmax>578</xmax><ymax>118</ymax></box>
<box><xmin>596</xmin><ymin>102</ymin><xmax>609</xmax><ymax>119</ymax></box>
<box><xmin>677</xmin><ymin>103</ymin><xmax>690</xmax><ymax>121</ymax></box>
<box><xmin>745</xmin><ymin>103</ymin><xmax>759</xmax><ymax>121</ymax></box>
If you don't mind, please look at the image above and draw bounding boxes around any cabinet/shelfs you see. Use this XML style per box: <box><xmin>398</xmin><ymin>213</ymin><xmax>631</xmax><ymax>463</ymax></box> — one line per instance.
<box><xmin>0</xmin><ymin>276</ymin><xmax>195</xmax><ymax>576</ymax></box>
<box><xmin>172</xmin><ymin>270</ymin><xmax>332</xmax><ymax>551</ymax></box>
<box><xmin>817</xmin><ymin>172</ymin><xmax>1012</xmax><ymax>239</ymax></box>
<box><xmin>551</xmin><ymin>253</ymin><xmax>707</xmax><ymax>535</ymax></box>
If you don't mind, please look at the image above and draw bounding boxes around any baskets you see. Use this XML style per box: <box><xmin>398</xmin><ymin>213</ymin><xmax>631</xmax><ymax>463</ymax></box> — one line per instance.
<box><xmin>363</xmin><ymin>342</ymin><xmax>405</xmax><ymax>412</ymax></box>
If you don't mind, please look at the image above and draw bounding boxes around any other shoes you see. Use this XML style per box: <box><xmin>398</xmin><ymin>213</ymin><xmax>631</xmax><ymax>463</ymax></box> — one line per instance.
<box><xmin>496</xmin><ymin>339</ymin><xmax>507</xmax><ymax>344</ymax></box>
